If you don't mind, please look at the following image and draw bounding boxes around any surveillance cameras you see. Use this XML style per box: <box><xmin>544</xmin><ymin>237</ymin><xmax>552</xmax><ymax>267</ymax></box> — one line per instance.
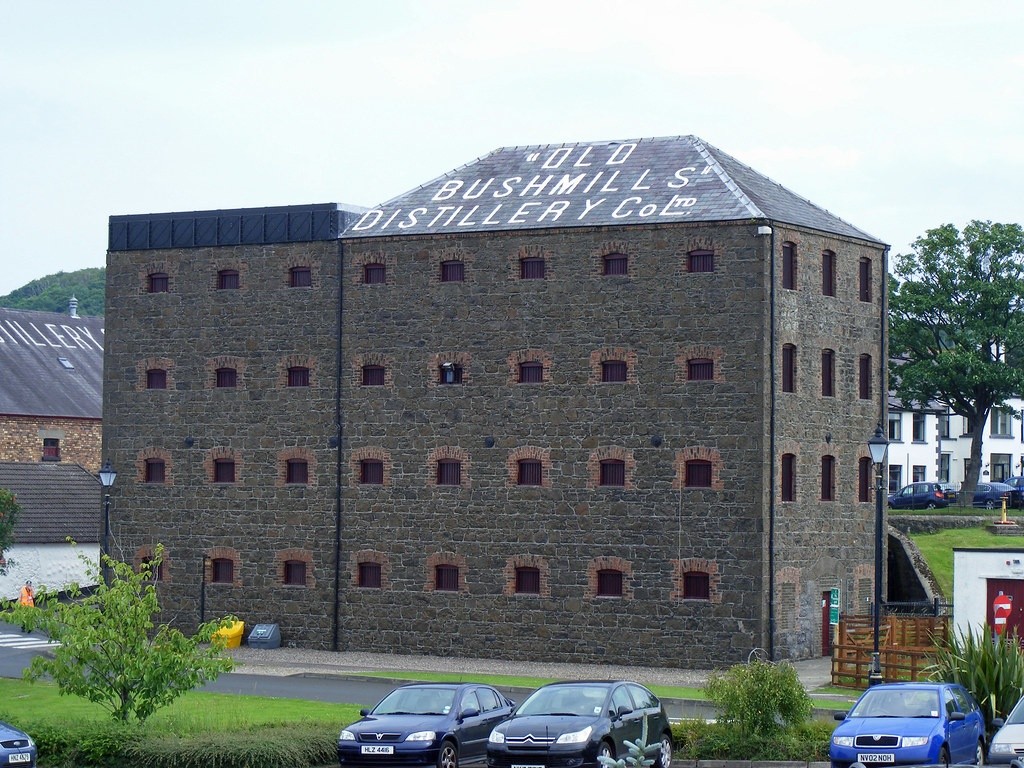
<box><xmin>763</xmin><ymin>226</ymin><xmax>773</xmax><ymax>236</ymax></box>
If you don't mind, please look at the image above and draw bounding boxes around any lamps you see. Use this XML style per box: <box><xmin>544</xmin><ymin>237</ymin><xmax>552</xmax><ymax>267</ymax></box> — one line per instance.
<box><xmin>441</xmin><ymin>362</ymin><xmax>455</xmax><ymax>374</ymax></box>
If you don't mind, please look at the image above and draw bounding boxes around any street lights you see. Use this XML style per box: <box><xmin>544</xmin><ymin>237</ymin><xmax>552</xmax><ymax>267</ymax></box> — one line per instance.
<box><xmin>99</xmin><ymin>456</ymin><xmax>117</xmax><ymax>624</ymax></box>
<box><xmin>867</xmin><ymin>419</ymin><xmax>892</xmax><ymax>687</ymax></box>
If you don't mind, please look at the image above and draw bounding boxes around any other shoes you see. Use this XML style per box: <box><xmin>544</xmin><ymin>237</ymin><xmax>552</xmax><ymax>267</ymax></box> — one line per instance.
<box><xmin>28</xmin><ymin>630</ymin><xmax>31</xmax><ymax>633</ymax></box>
<box><xmin>22</xmin><ymin>629</ymin><xmax>25</xmax><ymax>632</ymax></box>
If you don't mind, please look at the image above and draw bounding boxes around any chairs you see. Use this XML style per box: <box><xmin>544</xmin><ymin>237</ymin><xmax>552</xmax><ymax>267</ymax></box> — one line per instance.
<box><xmin>561</xmin><ymin>691</ymin><xmax>587</xmax><ymax>712</ymax></box>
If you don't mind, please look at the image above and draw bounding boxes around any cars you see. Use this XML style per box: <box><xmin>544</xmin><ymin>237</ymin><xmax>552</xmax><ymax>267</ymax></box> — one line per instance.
<box><xmin>942</xmin><ymin>482</ymin><xmax>1016</xmax><ymax>511</ymax></box>
<box><xmin>336</xmin><ymin>682</ymin><xmax>517</xmax><ymax>768</ymax></box>
<box><xmin>987</xmin><ymin>694</ymin><xmax>1024</xmax><ymax>768</ymax></box>
<box><xmin>487</xmin><ymin>680</ymin><xmax>677</xmax><ymax>768</ymax></box>
<box><xmin>888</xmin><ymin>480</ymin><xmax>957</xmax><ymax>511</ymax></box>
<box><xmin>0</xmin><ymin>721</ymin><xmax>38</xmax><ymax>768</ymax></box>
<box><xmin>826</xmin><ymin>683</ymin><xmax>988</xmax><ymax>766</ymax></box>
<box><xmin>1003</xmin><ymin>476</ymin><xmax>1024</xmax><ymax>505</ymax></box>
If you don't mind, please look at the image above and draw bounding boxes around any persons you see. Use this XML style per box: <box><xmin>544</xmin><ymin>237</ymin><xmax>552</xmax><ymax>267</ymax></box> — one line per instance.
<box><xmin>18</xmin><ymin>580</ymin><xmax>35</xmax><ymax>633</ymax></box>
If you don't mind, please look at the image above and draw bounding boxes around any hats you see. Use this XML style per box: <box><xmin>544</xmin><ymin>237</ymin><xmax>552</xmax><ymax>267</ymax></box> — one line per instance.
<box><xmin>25</xmin><ymin>580</ymin><xmax>32</xmax><ymax>585</ymax></box>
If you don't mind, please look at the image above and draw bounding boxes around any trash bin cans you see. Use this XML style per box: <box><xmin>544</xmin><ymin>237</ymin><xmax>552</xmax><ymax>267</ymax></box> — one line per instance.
<box><xmin>247</xmin><ymin>623</ymin><xmax>282</xmax><ymax>649</ymax></box>
<box><xmin>211</xmin><ymin>620</ymin><xmax>245</xmax><ymax>649</ymax></box>
